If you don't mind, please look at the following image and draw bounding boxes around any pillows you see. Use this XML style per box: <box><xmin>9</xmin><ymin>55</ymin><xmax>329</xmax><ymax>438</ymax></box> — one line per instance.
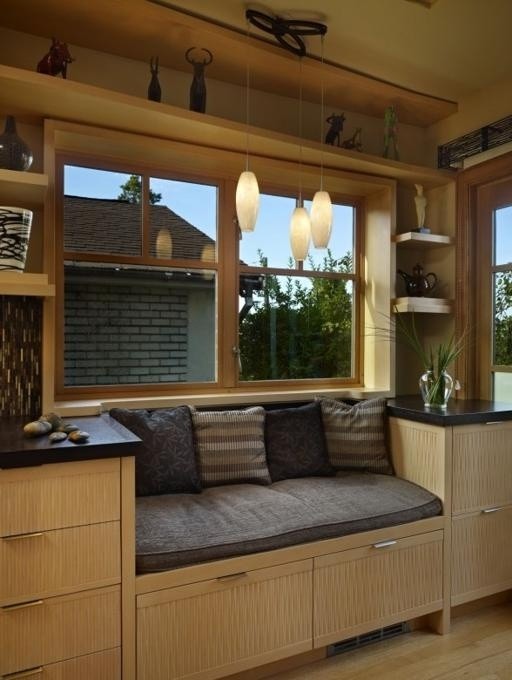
<box><xmin>191</xmin><ymin>406</ymin><xmax>273</xmax><ymax>486</ymax></box>
<box><xmin>314</xmin><ymin>394</ymin><xmax>395</xmax><ymax>475</ymax></box>
<box><xmin>109</xmin><ymin>406</ymin><xmax>202</xmax><ymax>498</ymax></box>
<box><xmin>264</xmin><ymin>400</ymin><xmax>334</xmax><ymax>483</ymax></box>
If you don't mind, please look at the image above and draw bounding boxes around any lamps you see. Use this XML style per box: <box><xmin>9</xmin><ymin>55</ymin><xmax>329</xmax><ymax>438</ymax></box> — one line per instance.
<box><xmin>310</xmin><ymin>28</ymin><xmax>334</xmax><ymax>248</ymax></box>
<box><xmin>201</xmin><ymin>244</ymin><xmax>216</xmax><ymax>279</ymax></box>
<box><xmin>236</xmin><ymin>19</ymin><xmax>259</xmax><ymax>232</ymax></box>
<box><xmin>156</xmin><ymin>213</ymin><xmax>173</xmax><ymax>261</ymax></box>
<box><xmin>290</xmin><ymin>56</ymin><xmax>312</xmax><ymax>262</ymax></box>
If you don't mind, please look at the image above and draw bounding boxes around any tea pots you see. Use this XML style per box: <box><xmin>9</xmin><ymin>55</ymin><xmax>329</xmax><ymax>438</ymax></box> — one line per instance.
<box><xmin>397</xmin><ymin>262</ymin><xmax>438</xmax><ymax>299</ymax></box>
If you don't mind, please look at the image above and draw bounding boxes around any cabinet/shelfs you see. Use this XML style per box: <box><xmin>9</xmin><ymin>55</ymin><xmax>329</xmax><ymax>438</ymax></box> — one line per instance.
<box><xmin>135</xmin><ymin>515</ymin><xmax>446</xmax><ymax>680</ymax></box>
<box><xmin>0</xmin><ymin>0</ymin><xmax>461</xmax><ymax>316</ymax></box>
<box><xmin>0</xmin><ymin>416</ymin><xmax>142</xmax><ymax>680</ymax></box>
<box><xmin>383</xmin><ymin>392</ymin><xmax>512</xmax><ymax>608</ymax></box>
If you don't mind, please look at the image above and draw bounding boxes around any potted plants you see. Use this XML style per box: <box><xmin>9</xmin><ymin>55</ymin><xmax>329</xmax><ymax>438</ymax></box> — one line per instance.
<box><xmin>356</xmin><ymin>303</ymin><xmax>479</xmax><ymax>409</ymax></box>
